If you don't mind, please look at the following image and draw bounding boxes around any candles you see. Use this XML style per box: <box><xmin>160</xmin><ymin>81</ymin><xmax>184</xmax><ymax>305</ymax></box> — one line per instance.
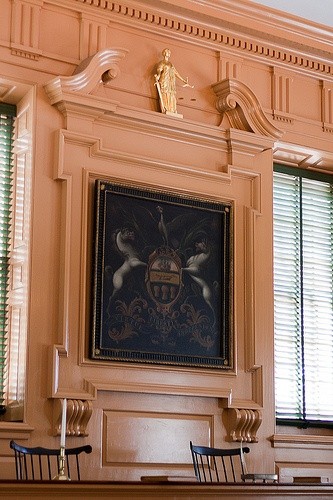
<box><xmin>61</xmin><ymin>398</ymin><xmax>67</xmax><ymax>451</ymax></box>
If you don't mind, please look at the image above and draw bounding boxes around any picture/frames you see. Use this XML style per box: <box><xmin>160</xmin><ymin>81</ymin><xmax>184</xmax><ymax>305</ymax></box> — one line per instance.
<box><xmin>89</xmin><ymin>174</ymin><xmax>237</xmax><ymax>370</ymax></box>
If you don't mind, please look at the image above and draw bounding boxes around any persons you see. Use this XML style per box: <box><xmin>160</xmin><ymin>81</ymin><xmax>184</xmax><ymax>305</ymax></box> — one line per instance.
<box><xmin>155</xmin><ymin>48</ymin><xmax>187</xmax><ymax>113</ymax></box>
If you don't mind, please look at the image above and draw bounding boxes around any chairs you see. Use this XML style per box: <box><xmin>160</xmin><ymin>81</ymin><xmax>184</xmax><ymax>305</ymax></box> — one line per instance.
<box><xmin>9</xmin><ymin>441</ymin><xmax>93</xmax><ymax>480</ymax></box>
<box><xmin>189</xmin><ymin>440</ymin><xmax>253</xmax><ymax>484</ymax></box>
